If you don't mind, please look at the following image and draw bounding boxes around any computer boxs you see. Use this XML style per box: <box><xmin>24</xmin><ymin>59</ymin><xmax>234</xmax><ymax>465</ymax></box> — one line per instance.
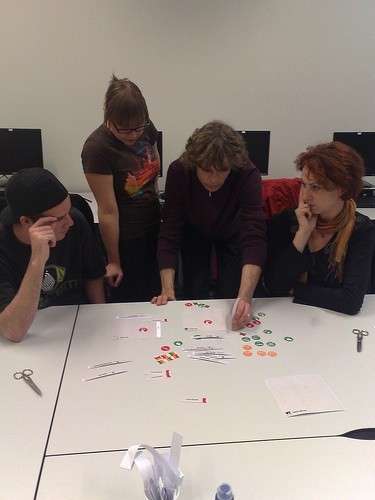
<box><xmin>355</xmin><ymin>188</ymin><xmax>375</xmax><ymax>208</ymax></box>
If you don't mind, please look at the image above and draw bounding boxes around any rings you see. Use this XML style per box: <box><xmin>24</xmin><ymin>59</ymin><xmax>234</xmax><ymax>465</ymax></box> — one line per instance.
<box><xmin>248</xmin><ymin>314</ymin><xmax>250</xmax><ymax>316</ymax></box>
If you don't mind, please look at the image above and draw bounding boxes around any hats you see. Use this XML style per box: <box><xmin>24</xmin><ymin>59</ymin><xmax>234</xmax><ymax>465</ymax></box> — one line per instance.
<box><xmin>0</xmin><ymin>167</ymin><xmax>68</xmax><ymax>228</ymax></box>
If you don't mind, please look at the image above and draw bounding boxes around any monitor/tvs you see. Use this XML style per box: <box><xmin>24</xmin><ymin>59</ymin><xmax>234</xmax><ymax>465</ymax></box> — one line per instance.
<box><xmin>0</xmin><ymin>128</ymin><xmax>44</xmax><ymax>176</ymax></box>
<box><xmin>157</xmin><ymin>130</ymin><xmax>163</xmax><ymax>176</ymax></box>
<box><xmin>334</xmin><ymin>131</ymin><xmax>375</xmax><ymax>188</ymax></box>
<box><xmin>235</xmin><ymin>131</ymin><xmax>271</xmax><ymax>176</ymax></box>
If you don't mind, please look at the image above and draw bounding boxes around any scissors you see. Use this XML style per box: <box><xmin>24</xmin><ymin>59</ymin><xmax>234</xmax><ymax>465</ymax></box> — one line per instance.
<box><xmin>353</xmin><ymin>329</ymin><xmax>368</xmax><ymax>352</ymax></box>
<box><xmin>14</xmin><ymin>369</ymin><xmax>42</xmax><ymax>396</ymax></box>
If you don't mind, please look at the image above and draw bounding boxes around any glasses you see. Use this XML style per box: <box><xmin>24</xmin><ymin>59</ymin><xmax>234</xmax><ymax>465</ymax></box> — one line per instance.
<box><xmin>112</xmin><ymin>120</ymin><xmax>148</xmax><ymax>134</ymax></box>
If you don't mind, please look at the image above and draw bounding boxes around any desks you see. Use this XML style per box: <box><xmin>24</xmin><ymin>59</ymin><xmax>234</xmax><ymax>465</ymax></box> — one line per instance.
<box><xmin>0</xmin><ymin>174</ymin><xmax>375</xmax><ymax>500</ymax></box>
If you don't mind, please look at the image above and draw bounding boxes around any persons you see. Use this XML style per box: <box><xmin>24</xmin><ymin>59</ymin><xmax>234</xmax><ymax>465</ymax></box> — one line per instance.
<box><xmin>262</xmin><ymin>142</ymin><xmax>375</xmax><ymax>314</ymax></box>
<box><xmin>0</xmin><ymin>168</ymin><xmax>107</xmax><ymax>342</ymax></box>
<box><xmin>151</xmin><ymin>120</ymin><xmax>266</xmax><ymax>330</ymax></box>
<box><xmin>80</xmin><ymin>78</ymin><xmax>161</xmax><ymax>302</ymax></box>
